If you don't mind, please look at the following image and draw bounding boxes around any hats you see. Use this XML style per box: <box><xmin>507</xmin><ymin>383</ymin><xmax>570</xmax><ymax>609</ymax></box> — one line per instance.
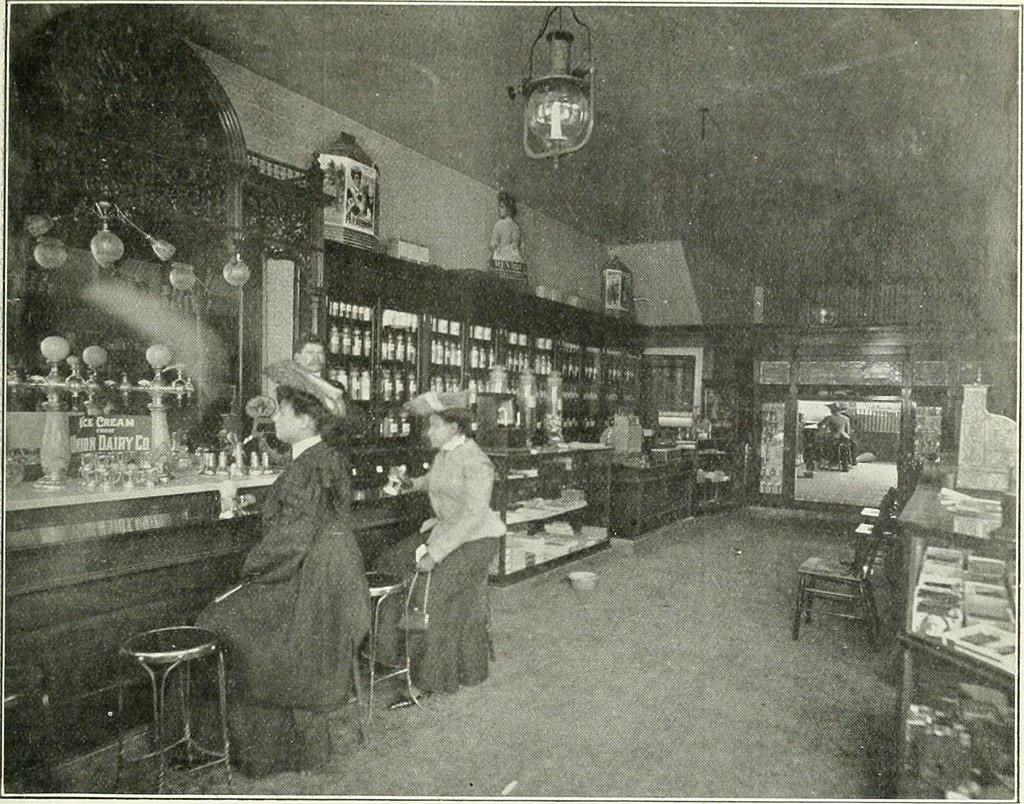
<box><xmin>402</xmin><ymin>391</ymin><xmax>471</xmax><ymax>418</ymax></box>
<box><xmin>263</xmin><ymin>355</ymin><xmax>349</xmax><ymax>418</ymax></box>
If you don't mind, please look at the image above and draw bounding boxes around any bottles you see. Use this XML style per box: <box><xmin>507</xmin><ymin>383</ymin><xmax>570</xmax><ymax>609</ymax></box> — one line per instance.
<box><xmin>321</xmin><ymin>297</ymin><xmax>645</xmax><ymax>458</ymax></box>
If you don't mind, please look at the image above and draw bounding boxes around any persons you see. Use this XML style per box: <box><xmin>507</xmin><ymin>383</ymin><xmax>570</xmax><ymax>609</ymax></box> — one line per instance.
<box><xmin>277</xmin><ymin>337</ymin><xmax>365</xmax><ymax>460</ymax></box>
<box><xmin>347</xmin><ymin>164</ymin><xmax>374</xmax><ymax>227</ymax></box>
<box><xmin>179</xmin><ymin>380</ymin><xmax>375</xmax><ymax>776</ymax></box>
<box><xmin>487</xmin><ymin>192</ymin><xmax>527</xmax><ymax>265</ymax></box>
<box><xmin>838</xmin><ymin>403</ymin><xmax>858</xmax><ymax>468</ymax></box>
<box><xmin>365</xmin><ymin>406</ymin><xmax>506</xmax><ymax>700</ymax></box>
<box><xmin>817</xmin><ymin>406</ymin><xmax>850</xmax><ymax>471</ymax></box>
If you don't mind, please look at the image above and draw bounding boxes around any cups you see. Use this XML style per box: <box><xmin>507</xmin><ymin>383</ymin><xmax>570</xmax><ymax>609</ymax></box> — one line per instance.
<box><xmin>78</xmin><ymin>447</ymin><xmax>175</xmax><ymax>491</ymax></box>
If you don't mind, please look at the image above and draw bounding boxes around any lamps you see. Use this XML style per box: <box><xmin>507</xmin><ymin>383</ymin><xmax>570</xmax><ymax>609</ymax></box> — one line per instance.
<box><xmin>507</xmin><ymin>7</ymin><xmax>596</xmax><ymax>171</ymax></box>
<box><xmin>165</xmin><ymin>214</ymin><xmax>259</xmax><ymax>293</ymax></box>
<box><xmin>24</xmin><ymin>152</ymin><xmax>175</xmax><ymax>273</ymax></box>
<box><xmin>119</xmin><ymin>340</ymin><xmax>192</xmax><ymax>472</ymax></box>
<box><xmin>10</xmin><ymin>333</ymin><xmax>106</xmax><ymax>484</ymax></box>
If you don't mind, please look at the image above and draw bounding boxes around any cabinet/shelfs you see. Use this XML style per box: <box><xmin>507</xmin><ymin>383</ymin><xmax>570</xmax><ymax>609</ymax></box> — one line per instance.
<box><xmin>321</xmin><ymin>237</ymin><xmax>642</xmax><ymax>439</ymax></box>
<box><xmin>899</xmin><ymin>527</ymin><xmax>1019</xmax><ymax>801</ymax></box>
<box><xmin>793</xmin><ymin>324</ymin><xmax>906</xmax><ymax>521</ymax></box>
<box><xmin>748</xmin><ymin>328</ymin><xmax>793</xmax><ymax>533</ymax></box>
<box><xmin>484</xmin><ymin>442</ymin><xmax>614</xmax><ymax>588</ymax></box>
<box><xmin>907</xmin><ymin>321</ymin><xmax>950</xmax><ymax>496</ymax></box>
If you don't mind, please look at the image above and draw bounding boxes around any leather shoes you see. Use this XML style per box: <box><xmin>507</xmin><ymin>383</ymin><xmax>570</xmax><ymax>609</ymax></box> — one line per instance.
<box><xmin>384</xmin><ymin>687</ymin><xmax>431</xmax><ymax>712</ymax></box>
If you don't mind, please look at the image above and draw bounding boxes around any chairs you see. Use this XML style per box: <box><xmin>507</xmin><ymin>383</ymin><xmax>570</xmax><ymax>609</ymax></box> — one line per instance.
<box><xmin>791</xmin><ymin>525</ymin><xmax>884</xmax><ymax>650</ymax></box>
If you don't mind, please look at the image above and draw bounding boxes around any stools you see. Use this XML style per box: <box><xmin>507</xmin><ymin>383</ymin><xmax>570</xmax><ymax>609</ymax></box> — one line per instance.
<box><xmin>354</xmin><ymin>566</ymin><xmax>424</xmax><ymax>721</ymax></box>
<box><xmin>103</xmin><ymin>626</ymin><xmax>236</xmax><ymax>796</ymax></box>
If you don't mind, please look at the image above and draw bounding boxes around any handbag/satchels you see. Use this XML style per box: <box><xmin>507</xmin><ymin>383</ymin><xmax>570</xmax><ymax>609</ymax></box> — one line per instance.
<box><xmin>397</xmin><ymin>609</ymin><xmax>430</xmax><ymax>631</ymax></box>
